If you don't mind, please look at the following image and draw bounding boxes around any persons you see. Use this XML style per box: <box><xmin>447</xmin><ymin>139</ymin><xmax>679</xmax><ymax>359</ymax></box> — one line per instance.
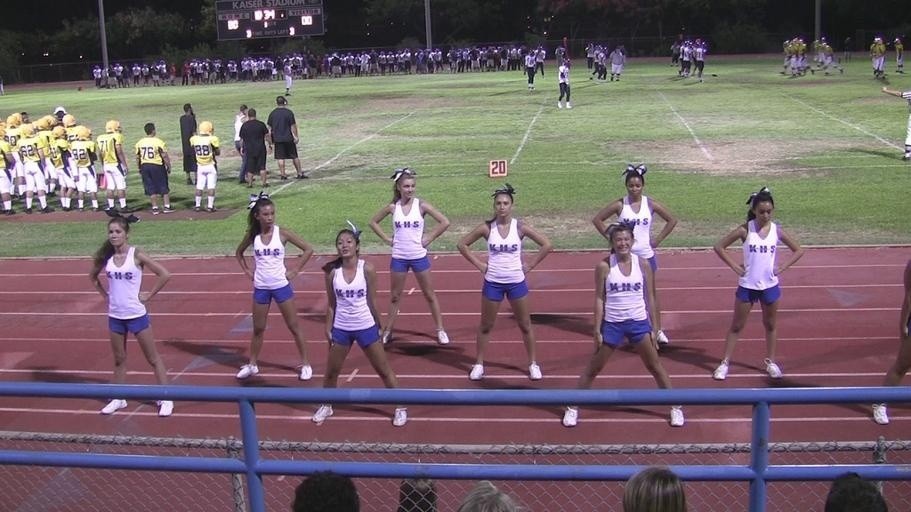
<box><xmin>824</xmin><ymin>471</ymin><xmax>888</xmax><ymax>512</ymax></box>
<box><xmin>234</xmin><ymin>96</ymin><xmax>310</xmax><ymax>190</ymax></box>
<box><xmin>447</xmin><ymin>46</ymin><xmax>529</xmax><ymax>74</ymax></box>
<box><xmin>290</xmin><ymin>468</ymin><xmax>361</xmax><ymax>511</ymax></box>
<box><xmin>592</xmin><ymin>162</ymin><xmax>677</xmax><ymax>343</ymax></box>
<box><xmin>94</xmin><ymin>60</ymin><xmax>177</xmax><ymax>90</ymax></box>
<box><xmin>134</xmin><ymin>124</ymin><xmax>175</xmax><ymax>215</ymax></box>
<box><xmin>668</xmin><ymin>36</ymin><xmax>712</xmax><ymax>85</ymax></box>
<box><xmin>711</xmin><ymin>187</ymin><xmax>804</xmax><ymax>381</ymax></box>
<box><xmin>524</xmin><ymin>47</ymin><xmax>546</xmax><ymax>92</ymax></box>
<box><xmin>309</xmin><ymin>230</ymin><xmax>408</xmax><ymax>428</ymax></box>
<box><xmin>89</xmin><ymin>209</ymin><xmax>175</xmax><ymax>417</ymax></box>
<box><xmin>236</xmin><ymin>191</ymin><xmax>313</xmax><ymax>384</ymax></box>
<box><xmin>190</xmin><ymin>121</ymin><xmax>221</xmax><ymax>211</ymax></box>
<box><xmin>621</xmin><ymin>463</ymin><xmax>687</xmax><ymax>512</ymax></box>
<box><xmin>555</xmin><ymin>44</ymin><xmax>572</xmax><ymax>108</ymax></box>
<box><xmin>0</xmin><ymin>107</ymin><xmax>129</xmax><ymax>212</ymax></box>
<box><xmin>882</xmin><ymin>86</ymin><xmax>911</xmax><ymax>161</ymax></box>
<box><xmin>395</xmin><ymin>478</ymin><xmax>436</xmax><ymax>512</ymax></box>
<box><xmin>368</xmin><ymin>168</ymin><xmax>453</xmax><ymax>347</ymax></box>
<box><xmin>283</xmin><ymin>60</ymin><xmax>293</xmax><ymax>96</ymax></box>
<box><xmin>872</xmin><ymin>259</ymin><xmax>911</xmax><ymax>425</ymax></box>
<box><xmin>454</xmin><ymin>480</ymin><xmax>516</xmax><ymax>512</ymax></box>
<box><xmin>182</xmin><ymin>55</ymin><xmax>293</xmax><ymax>85</ymax></box>
<box><xmin>778</xmin><ymin>32</ymin><xmax>904</xmax><ymax>78</ymax></box>
<box><xmin>456</xmin><ymin>184</ymin><xmax>552</xmax><ymax>380</ymax></box>
<box><xmin>560</xmin><ymin>220</ymin><xmax>685</xmax><ymax>428</ymax></box>
<box><xmin>180</xmin><ymin>103</ymin><xmax>198</xmax><ymax>185</ymax></box>
<box><xmin>0</xmin><ymin>76</ymin><xmax>5</xmax><ymax>96</ymax></box>
<box><xmin>585</xmin><ymin>43</ymin><xmax>625</xmax><ymax>81</ymax></box>
<box><xmin>292</xmin><ymin>48</ymin><xmax>444</xmax><ymax>80</ymax></box>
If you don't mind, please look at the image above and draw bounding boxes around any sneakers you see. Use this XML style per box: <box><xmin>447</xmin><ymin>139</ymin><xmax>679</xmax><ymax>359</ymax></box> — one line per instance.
<box><xmin>469</xmin><ymin>364</ymin><xmax>484</xmax><ymax>380</ymax></box>
<box><xmin>207</xmin><ymin>206</ymin><xmax>217</xmax><ymax>212</ymax></box>
<box><xmin>236</xmin><ymin>364</ymin><xmax>259</xmax><ymax>379</ymax></box>
<box><xmin>382</xmin><ymin>330</ymin><xmax>393</xmax><ymax>344</ymax></box>
<box><xmin>297</xmin><ymin>172</ymin><xmax>308</xmax><ymax>179</ymax></box>
<box><xmin>281</xmin><ymin>176</ymin><xmax>287</xmax><ymax>180</ymax></box>
<box><xmin>766</xmin><ymin>362</ymin><xmax>783</xmax><ymax>378</ymax></box>
<box><xmin>121</xmin><ymin>206</ymin><xmax>133</xmax><ymax>213</ymax></box>
<box><xmin>656</xmin><ymin>329</ymin><xmax>668</xmax><ymax>344</ymax></box>
<box><xmin>872</xmin><ymin>403</ymin><xmax>889</xmax><ymax>425</ymax></box>
<box><xmin>713</xmin><ymin>364</ymin><xmax>728</xmax><ymax>380</ymax></box>
<box><xmin>102</xmin><ymin>399</ymin><xmax>128</xmax><ymax>414</ymax></box>
<box><xmin>437</xmin><ymin>330</ymin><xmax>449</xmax><ymax>345</ymax></box>
<box><xmin>163</xmin><ymin>207</ymin><xmax>176</xmax><ymax>213</ymax></box>
<box><xmin>313</xmin><ymin>404</ymin><xmax>333</xmax><ymax>423</ymax></box>
<box><xmin>193</xmin><ymin>206</ymin><xmax>200</xmax><ymax>212</ymax></box>
<box><xmin>152</xmin><ymin>207</ymin><xmax>160</xmax><ymax>215</ymax></box>
<box><xmin>300</xmin><ymin>365</ymin><xmax>313</xmax><ymax>381</ymax></box>
<box><xmin>670</xmin><ymin>406</ymin><xmax>684</xmax><ymax>426</ymax></box>
<box><xmin>1</xmin><ymin>206</ymin><xmax>57</xmax><ymax>214</ymax></box>
<box><xmin>562</xmin><ymin>405</ymin><xmax>578</xmax><ymax>427</ymax></box>
<box><xmin>529</xmin><ymin>365</ymin><xmax>542</xmax><ymax>380</ymax></box>
<box><xmin>393</xmin><ymin>407</ymin><xmax>408</xmax><ymax>426</ymax></box>
<box><xmin>158</xmin><ymin>400</ymin><xmax>174</xmax><ymax>417</ymax></box>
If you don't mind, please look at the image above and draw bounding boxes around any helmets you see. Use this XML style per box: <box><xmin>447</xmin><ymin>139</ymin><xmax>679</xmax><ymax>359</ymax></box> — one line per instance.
<box><xmin>106</xmin><ymin>120</ymin><xmax>120</xmax><ymax>132</ymax></box>
<box><xmin>199</xmin><ymin>121</ymin><xmax>212</xmax><ymax>133</ymax></box>
<box><xmin>0</xmin><ymin>113</ymin><xmax>91</xmax><ymax>140</ymax></box>
